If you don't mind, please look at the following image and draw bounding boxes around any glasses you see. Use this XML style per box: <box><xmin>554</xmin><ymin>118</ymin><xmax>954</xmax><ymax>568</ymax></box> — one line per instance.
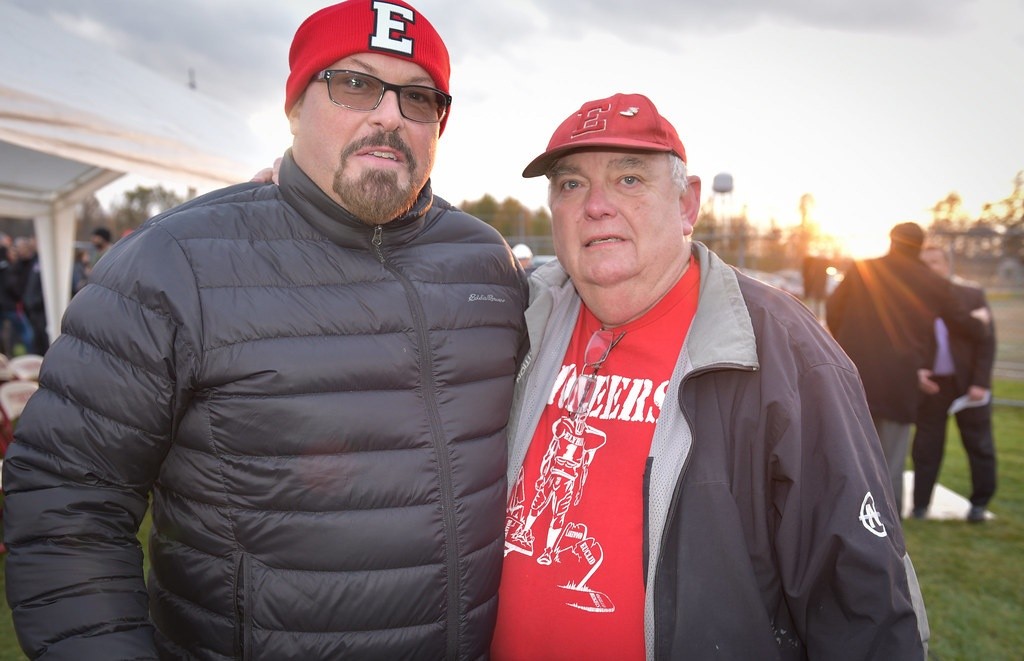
<box><xmin>312</xmin><ymin>70</ymin><xmax>452</xmax><ymax>124</ymax></box>
<box><xmin>92</xmin><ymin>241</ymin><xmax>105</xmax><ymax>251</ymax></box>
<box><xmin>561</xmin><ymin>329</ymin><xmax>629</xmax><ymax>419</ymax></box>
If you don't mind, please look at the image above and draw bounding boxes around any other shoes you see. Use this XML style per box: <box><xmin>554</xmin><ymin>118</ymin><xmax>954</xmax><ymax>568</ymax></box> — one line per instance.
<box><xmin>911</xmin><ymin>509</ymin><xmax>926</xmax><ymax>521</ymax></box>
<box><xmin>968</xmin><ymin>506</ymin><xmax>997</xmax><ymax>522</ymax></box>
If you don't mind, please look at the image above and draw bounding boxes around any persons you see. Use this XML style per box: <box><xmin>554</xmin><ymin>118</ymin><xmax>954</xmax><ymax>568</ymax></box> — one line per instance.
<box><xmin>511</xmin><ymin>243</ymin><xmax>537</xmax><ymax>276</ymax></box>
<box><xmin>0</xmin><ymin>227</ymin><xmax>133</xmax><ymax>359</ymax></box>
<box><xmin>826</xmin><ymin>222</ymin><xmax>997</xmax><ymax>523</ymax></box>
<box><xmin>249</xmin><ymin>92</ymin><xmax>930</xmax><ymax>661</ymax></box>
<box><xmin>0</xmin><ymin>0</ymin><xmax>528</xmax><ymax>661</ymax></box>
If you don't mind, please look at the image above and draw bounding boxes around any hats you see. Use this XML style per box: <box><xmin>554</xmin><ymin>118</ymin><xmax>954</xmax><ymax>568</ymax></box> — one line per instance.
<box><xmin>93</xmin><ymin>227</ymin><xmax>112</xmax><ymax>243</ymax></box>
<box><xmin>285</xmin><ymin>0</ymin><xmax>451</xmax><ymax>137</ymax></box>
<box><xmin>523</xmin><ymin>92</ymin><xmax>687</xmax><ymax>177</ymax></box>
